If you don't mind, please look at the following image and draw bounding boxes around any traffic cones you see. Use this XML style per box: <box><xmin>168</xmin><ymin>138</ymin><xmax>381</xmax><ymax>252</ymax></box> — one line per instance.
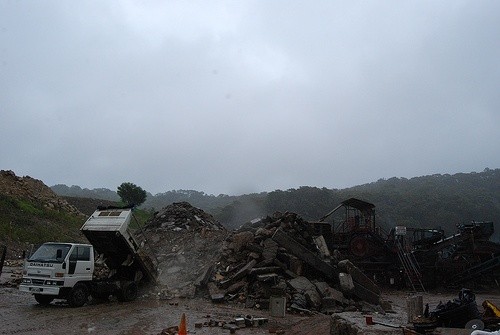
<box><xmin>177</xmin><ymin>313</ymin><xmax>190</xmax><ymax>335</ymax></box>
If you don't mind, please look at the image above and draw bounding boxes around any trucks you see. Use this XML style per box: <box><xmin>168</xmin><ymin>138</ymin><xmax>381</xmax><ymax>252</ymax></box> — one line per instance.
<box><xmin>20</xmin><ymin>205</ymin><xmax>162</xmax><ymax>307</ymax></box>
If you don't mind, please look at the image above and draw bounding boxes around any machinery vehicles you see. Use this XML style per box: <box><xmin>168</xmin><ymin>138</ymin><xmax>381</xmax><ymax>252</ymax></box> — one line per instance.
<box><xmin>334</xmin><ymin>198</ymin><xmax>494</xmax><ymax>288</ymax></box>
